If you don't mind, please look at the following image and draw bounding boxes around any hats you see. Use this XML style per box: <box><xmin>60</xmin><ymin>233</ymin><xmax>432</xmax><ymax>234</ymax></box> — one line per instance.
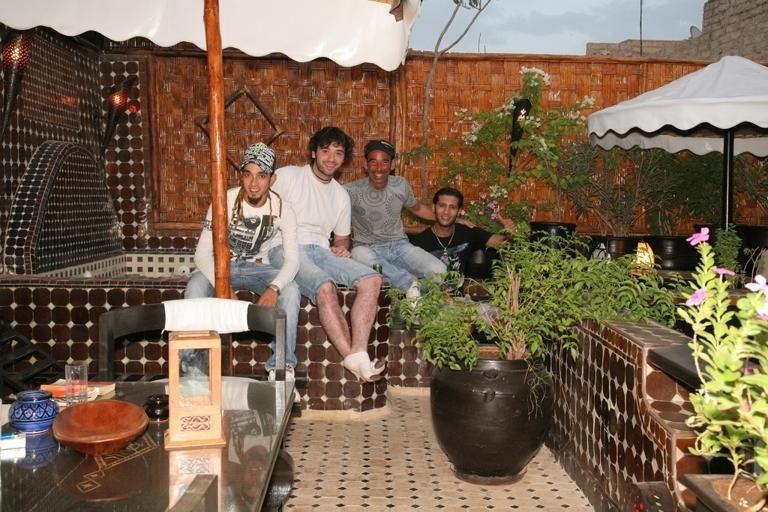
<box><xmin>364</xmin><ymin>139</ymin><xmax>394</xmax><ymax>158</ymax></box>
<box><xmin>238</xmin><ymin>142</ymin><xmax>275</xmax><ymax>175</ymax></box>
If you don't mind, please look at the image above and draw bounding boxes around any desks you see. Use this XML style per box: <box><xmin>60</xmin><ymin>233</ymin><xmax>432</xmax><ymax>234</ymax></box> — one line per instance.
<box><xmin>0</xmin><ymin>376</ymin><xmax>299</xmax><ymax>512</ymax></box>
<box><xmin>631</xmin><ymin>268</ymin><xmax>709</xmax><ymax>286</ymax></box>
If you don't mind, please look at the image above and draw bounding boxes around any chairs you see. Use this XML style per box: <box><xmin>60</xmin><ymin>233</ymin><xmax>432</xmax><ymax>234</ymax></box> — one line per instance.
<box><xmin>97</xmin><ymin>297</ymin><xmax>294</xmax><ymax>512</ymax></box>
<box><xmin>0</xmin><ymin>327</ymin><xmax>65</xmax><ymax>400</ymax></box>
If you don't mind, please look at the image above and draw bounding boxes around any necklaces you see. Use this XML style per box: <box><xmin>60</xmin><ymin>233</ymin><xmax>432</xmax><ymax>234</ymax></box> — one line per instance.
<box><xmin>433</xmin><ymin>224</ymin><xmax>455</xmax><ymax>260</ymax></box>
<box><xmin>313</xmin><ymin>171</ymin><xmax>332</xmax><ymax>183</ymax></box>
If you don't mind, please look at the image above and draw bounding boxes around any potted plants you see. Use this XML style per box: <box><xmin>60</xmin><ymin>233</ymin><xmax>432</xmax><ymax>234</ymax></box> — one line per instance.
<box><xmin>693</xmin><ymin>153</ymin><xmax>732</xmax><ymax>244</ymax></box>
<box><xmin>385</xmin><ymin>203</ymin><xmax>677</xmax><ymax>476</ymax></box>
<box><xmin>569</xmin><ymin>131</ymin><xmax>655</xmax><ymax>263</ymax></box>
<box><xmin>735</xmin><ymin>155</ymin><xmax>768</xmax><ymax>277</ymax></box>
<box><xmin>643</xmin><ymin>148</ymin><xmax>692</xmax><ymax>269</ymax></box>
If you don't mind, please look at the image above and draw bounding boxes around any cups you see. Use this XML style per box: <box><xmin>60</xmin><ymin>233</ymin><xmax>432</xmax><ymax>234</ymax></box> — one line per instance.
<box><xmin>65</xmin><ymin>364</ymin><xmax>88</xmax><ymax>405</ymax></box>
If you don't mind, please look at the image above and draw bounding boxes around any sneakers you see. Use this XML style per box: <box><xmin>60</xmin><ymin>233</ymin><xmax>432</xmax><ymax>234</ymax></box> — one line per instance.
<box><xmin>268</xmin><ymin>362</ymin><xmax>301</xmax><ymax>403</ymax></box>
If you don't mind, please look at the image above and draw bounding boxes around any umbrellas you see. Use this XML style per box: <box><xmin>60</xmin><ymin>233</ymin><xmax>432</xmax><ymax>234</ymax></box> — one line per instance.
<box><xmin>1</xmin><ymin>1</ymin><xmax>422</xmax><ymax>379</ymax></box>
<box><xmin>587</xmin><ymin>55</ymin><xmax>768</xmax><ymax>273</ymax></box>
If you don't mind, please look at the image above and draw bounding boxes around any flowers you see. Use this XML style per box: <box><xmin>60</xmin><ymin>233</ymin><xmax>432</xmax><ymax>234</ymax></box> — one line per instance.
<box><xmin>460</xmin><ymin>185</ymin><xmax>516</xmax><ymax>233</ymax></box>
<box><xmin>678</xmin><ymin>227</ymin><xmax>768</xmax><ymax>500</ymax></box>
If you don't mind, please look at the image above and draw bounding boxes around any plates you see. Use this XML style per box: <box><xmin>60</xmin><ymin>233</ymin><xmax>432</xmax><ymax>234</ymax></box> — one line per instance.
<box><xmin>52</xmin><ymin>400</ymin><xmax>149</xmax><ymax>455</ymax></box>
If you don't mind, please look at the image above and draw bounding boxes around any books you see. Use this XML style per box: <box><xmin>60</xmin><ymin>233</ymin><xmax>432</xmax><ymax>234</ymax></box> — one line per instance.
<box><xmin>39</xmin><ymin>379</ymin><xmax>116</xmax><ymax>407</ymax></box>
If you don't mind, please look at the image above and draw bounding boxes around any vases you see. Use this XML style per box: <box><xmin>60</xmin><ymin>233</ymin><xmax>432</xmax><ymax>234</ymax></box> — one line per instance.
<box><xmin>464</xmin><ymin>233</ymin><xmax>495</xmax><ymax>274</ymax></box>
<box><xmin>684</xmin><ymin>474</ymin><xmax>768</xmax><ymax>512</ymax></box>
<box><xmin>529</xmin><ymin>223</ymin><xmax>576</xmax><ymax>260</ymax></box>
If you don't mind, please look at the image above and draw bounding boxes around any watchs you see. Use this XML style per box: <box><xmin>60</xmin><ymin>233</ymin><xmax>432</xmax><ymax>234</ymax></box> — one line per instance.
<box><xmin>267</xmin><ymin>284</ymin><xmax>281</xmax><ymax>296</ymax></box>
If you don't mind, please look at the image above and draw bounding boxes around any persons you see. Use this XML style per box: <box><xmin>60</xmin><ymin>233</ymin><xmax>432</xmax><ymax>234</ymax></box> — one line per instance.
<box><xmin>221</xmin><ymin>382</ymin><xmax>277</xmax><ymax>506</ymax></box>
<box><xmin>179</xmin><ymin>142</ymin><xmax>301</xmax><ymax>404</ymax></box>
<box><xmin>340</xmin><ymin>140</ymin><xmax>477</xmax><ymax>326</ymax></box>
<box><xmin>406</xmin><ymin>187</ymin><xmax>518</xmax><ymax>337</ymax></box>
<box><xmin>269</xmin><ymin>126</ymin><xmax>387</xmax><ymax>383</ymax></box>
